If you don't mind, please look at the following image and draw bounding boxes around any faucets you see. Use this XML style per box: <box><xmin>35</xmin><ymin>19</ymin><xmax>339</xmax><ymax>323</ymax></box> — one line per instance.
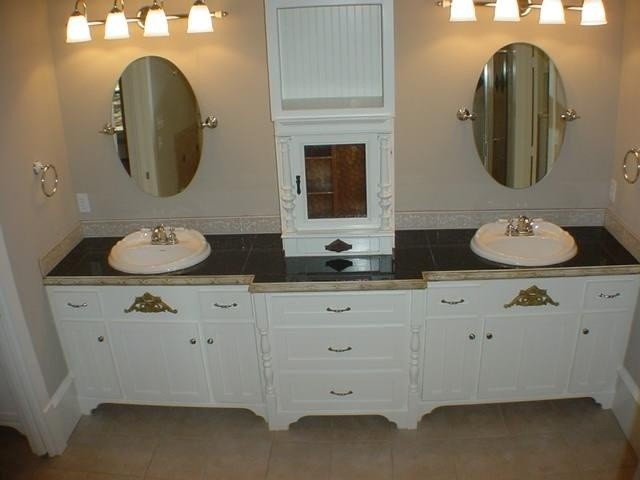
<box><xmin>516</xmin><ymin>216</ymin><xmax>531</xmax><ymax>235</ymax></box>
<box><xmin>152</xmin><ymin>226</ymin><xmax>167</xmax><ymax>243</ymax></box>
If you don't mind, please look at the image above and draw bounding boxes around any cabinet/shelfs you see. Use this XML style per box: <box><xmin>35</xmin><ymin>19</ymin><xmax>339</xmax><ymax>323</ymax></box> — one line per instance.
<box><xmin>51</xmin><ymin>290</ymin><xmax>124</xmax><ymax>400</ymax></box>
<box><xmin>418</xmin><ymin>273</ymin><xmax>581</xmax><ymax>423</ymax></box>
<box><xmin>567</xmin><ymin>274</ymin><xmax>640</xmax><ymax>395</ymax></box>
<box><xmin>258</xmin><ymin>281</ymin><xmax>419</xmax><ymax>432</ymax></box>
<box><xmin>96</xmin><ymin>284</ymin><xmax>263</xmax><ymax>405</ymax></box>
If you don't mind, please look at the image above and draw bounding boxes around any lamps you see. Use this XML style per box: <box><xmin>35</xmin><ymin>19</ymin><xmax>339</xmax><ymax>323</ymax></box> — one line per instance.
<box><xmin>433</xmin><ymin>0</ymin><xmax>608</xmax><ymax>26</ymax></box>
<box><xmin>63</xmin><ymin>0</ymin><xmax>229</xmax><ymax>43</ymax></box>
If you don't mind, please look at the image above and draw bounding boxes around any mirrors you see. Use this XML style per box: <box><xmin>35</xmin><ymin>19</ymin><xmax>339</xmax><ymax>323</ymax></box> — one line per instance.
<box><xmin>111</xmin><ymin>55</ymin><xmax>204</xmax><ymax>199</ymax></box>
<box><xmin>471</xmin><ymin>41</ymin><xmax>568</xmax><ymax>190</ymax></box>
<box><xmin>288</xmin><ymin>132</ymin><xmax>383</xmax><ymax>231</ymax></box>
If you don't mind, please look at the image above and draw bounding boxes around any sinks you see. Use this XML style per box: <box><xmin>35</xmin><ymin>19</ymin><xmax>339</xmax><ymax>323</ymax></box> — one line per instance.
<box><xmin>471</xmin><ymin>221</ymin><xmax>578</xmax><ymax>265</ymax></box>
<box><xmin>107</xmin><ymin>228</ymin><xmax>212</xmax><ymax>274</ymax></box>
